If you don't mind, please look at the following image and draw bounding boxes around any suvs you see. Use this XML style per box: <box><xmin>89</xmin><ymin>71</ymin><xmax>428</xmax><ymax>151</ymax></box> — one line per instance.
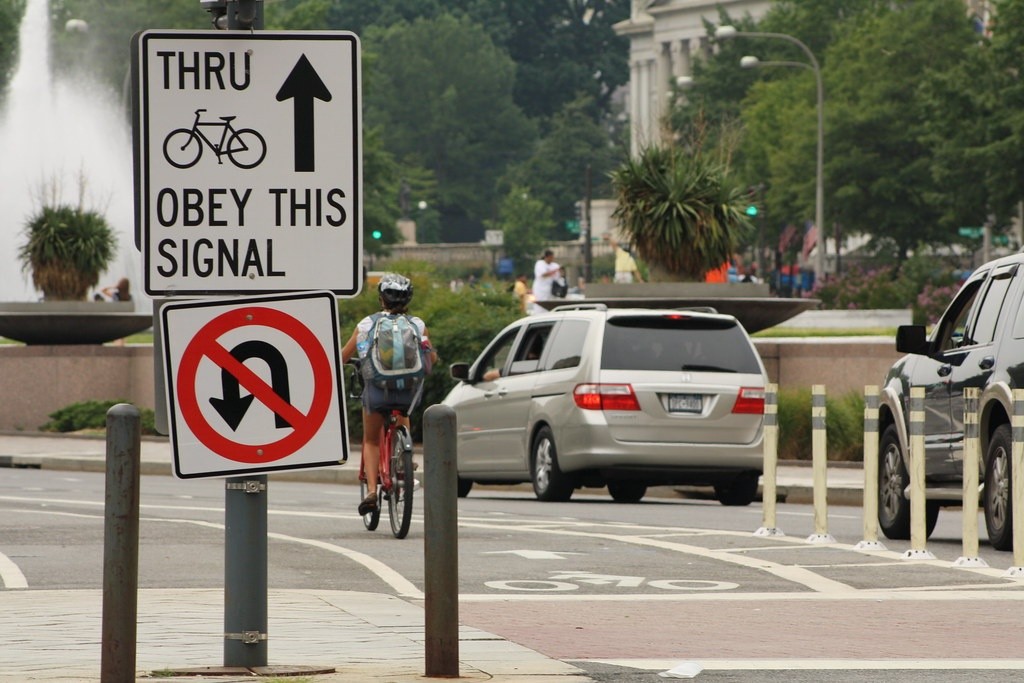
<box><xmin>436</xmin><ymin>302</ymin><xmax>772</xmax><ymax>508</ymax></box>
<box><xmin>877</xmin><ymin>249</ymin><xmax>1023</xmax><ymax>552</ymax></box>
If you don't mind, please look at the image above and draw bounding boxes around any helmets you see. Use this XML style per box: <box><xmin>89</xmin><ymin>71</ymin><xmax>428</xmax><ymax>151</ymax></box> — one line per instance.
<box><xmin>378</xmin><ymin>273</ymin><xmax>414</xmax><ymax>305</ymax></box>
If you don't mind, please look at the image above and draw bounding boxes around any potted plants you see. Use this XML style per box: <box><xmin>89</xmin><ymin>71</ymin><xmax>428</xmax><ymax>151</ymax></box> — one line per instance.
<box><xmin>15</xmin><ymin>163</ymin><xmax>122</xmax><ymax>303</ymax></box>
<box><xmin>603</xmin><ymin>101</ymin><xmax>764</xmax><ymax>285</ymax></box>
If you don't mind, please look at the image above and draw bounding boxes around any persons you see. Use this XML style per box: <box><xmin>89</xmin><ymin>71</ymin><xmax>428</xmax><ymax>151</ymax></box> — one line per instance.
<box><xmin>608</xmin><ymin>237</ymin><xmax>644</xmax><ymax>283</ymax></box>
<box><xmin>741</xmin><ymin>264</ymin><xmax>755</xmax><ymax>282</ymax></box>
<box><xmin>483</xmin><ymin>333</ymin><xmax>544</xmax><ymax>381</ymax></box>
<box><xmin>103</xmin><ymin>278</ymin><xmax>131</xmax><ymax>345</ymax></box>
<box><xmin>513</xmin><ymin>272</ymin><xmax>533</xmax><ymax>301</ymax></box>
<box><xmin>552</xmin><ymin>267</ymin><xmax>568</xmax><ymax>297</ymax></box>
<box><xmin>342</xmin><ymin>273</ymin><xmax>438</xmax><ymax>516</ymax></box>
<box><xmin>468</xmin><ymin>274</ymin><xmax>477</xmax><ymax>289</ymax></box>
<box><xmin>726</xmin><ymin>257</ymin><xmax>738</xmax><ymax>281</ymax></box>
<box><xmin>532</xmin><ymin>249</ymin><xmax>565</xmax><ymax>299</ymax></box>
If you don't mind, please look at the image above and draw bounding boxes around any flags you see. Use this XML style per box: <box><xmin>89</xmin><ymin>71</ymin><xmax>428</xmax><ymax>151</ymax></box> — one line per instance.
<box><xmin>803</xmin><ymin>220</ymin><xmax>818</xmax><ymax>260</ymax></box>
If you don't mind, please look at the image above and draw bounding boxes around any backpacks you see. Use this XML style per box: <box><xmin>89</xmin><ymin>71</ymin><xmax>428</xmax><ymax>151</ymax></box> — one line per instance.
<box><xmin>368</xmin><ymin>311</ymin><xmax>424</xmax><ymax>390</ymax></box>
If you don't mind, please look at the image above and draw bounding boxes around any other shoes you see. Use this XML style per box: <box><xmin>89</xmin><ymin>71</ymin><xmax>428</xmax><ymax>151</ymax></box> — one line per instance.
<box><xmin>358</xmin><ymin>491</ymin><xmax>379</xmax><ymax>516</ymax></box>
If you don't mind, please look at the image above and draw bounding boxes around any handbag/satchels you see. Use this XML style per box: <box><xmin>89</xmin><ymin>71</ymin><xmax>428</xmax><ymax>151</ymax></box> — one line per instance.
<box><xmin>551</xmin><ymin>267</ymin><xmax>568</xmax><ymax>298</ymax></box>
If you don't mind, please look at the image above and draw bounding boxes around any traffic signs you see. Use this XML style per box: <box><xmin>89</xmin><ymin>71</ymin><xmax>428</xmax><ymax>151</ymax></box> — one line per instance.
<box><xmin>136</xmin><ymin>25</ymin><xmax>366</xmax><ymax>302</ymax></box>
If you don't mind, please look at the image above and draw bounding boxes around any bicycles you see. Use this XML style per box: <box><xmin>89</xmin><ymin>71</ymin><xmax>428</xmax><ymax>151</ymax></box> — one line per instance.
<box><xmin>345</xmin><ymin>356</ymin><xmax>435</xmax><ymax>539</ymax></box>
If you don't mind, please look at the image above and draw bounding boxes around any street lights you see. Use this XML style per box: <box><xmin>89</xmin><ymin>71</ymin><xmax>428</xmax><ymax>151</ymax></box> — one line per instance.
<box><xmin>714</xmin><ymin>24</ymin><xmax>826</xmax><ymax>298</ymax></box>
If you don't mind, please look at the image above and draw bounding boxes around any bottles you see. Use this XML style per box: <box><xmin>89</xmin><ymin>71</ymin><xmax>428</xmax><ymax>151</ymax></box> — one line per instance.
<box><xmin>357</xmin><ymin>333</ymin><xmax>370</xmax><ymax>359</ymax></box>
<box><xmin>421</xmin><ymin>335</ymin><xmax>431</xmax><ymax>350</ymax></box>
